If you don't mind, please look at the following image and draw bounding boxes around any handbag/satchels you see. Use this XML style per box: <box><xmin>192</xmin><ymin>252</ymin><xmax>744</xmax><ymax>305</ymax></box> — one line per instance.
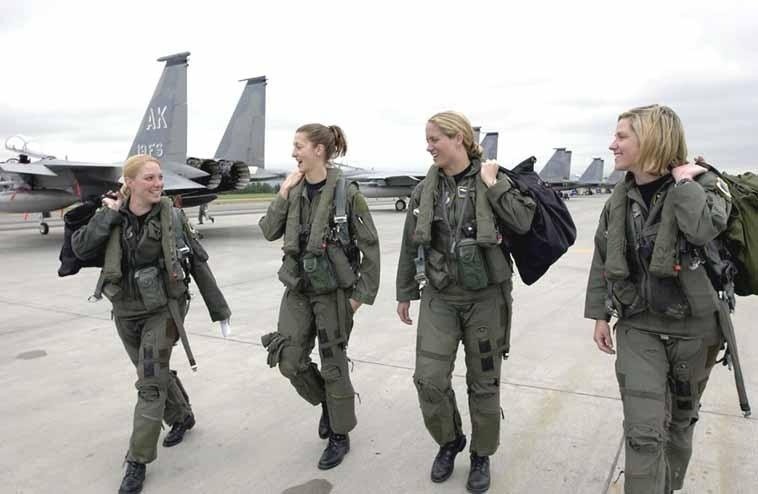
<box><xmin>679</xmin><ymin>253</ymin><xmax>721</xmax><ymax>317</ymax></box>
<box><xmin>695</xmin><ymin>161</ymin><xmax>758</xmax><ymax>296</ymax></box>
<box><xmin>58</xmin><ymin>193</ymin><xmax>117</xmax><ymax>277</ymax></box>
<box><xmin>134</xmin><ymin>266</ymin><xmax>167</xmax><ymax>311</ymax></box>
<box><xmin>498</xmin><ymin>156</ymin><xmax>576</xmax><ymax>286</ymax></box>
<box><xmin>458</xmin><ymin>238</ymin><xmax>488</xmax><ymax>290</ymax></box>
<box><xmin>304</xmin><ymin>255</ymin><xmax>338</xmax><ymax>295</ymax></box>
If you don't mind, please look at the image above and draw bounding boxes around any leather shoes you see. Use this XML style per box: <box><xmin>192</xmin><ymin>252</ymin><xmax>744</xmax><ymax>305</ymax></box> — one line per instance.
<box><xmin>163</xmin><ymin>413</ymin><xmax>195</xmax><ymax>447</ymax></box>
<box><xmin>430</xmin><ymin>435</ymin><xmax>466</xmax><ymax>483</ymax></box>
<box><xmin>318</xmin><ymin>400</ymin><xmax>350</xmax><ymax>469</ymax></box>
<box><xmin>118</xmin><ymin>461</ymin><xmax>146</xmax><ymax>494</ymax></box>
<box><xmin>467</xmin><ymin>453</ymin><xmax>490</xmax><ymax>494</ymax></box>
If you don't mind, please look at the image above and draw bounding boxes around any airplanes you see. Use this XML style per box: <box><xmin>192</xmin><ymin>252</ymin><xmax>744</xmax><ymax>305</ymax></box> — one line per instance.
<box><xmin>0</xmin><ymin>51</ymin><xmax>286</xmax><ymax>235</ymax></box>
<box><xmin>329</xmin><ymin>127</ymin><xmax>627</xmax><ymax>211</ymax></box>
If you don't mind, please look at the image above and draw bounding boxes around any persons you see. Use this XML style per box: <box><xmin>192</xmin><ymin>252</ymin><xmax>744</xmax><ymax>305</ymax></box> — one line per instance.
<box><xmin>198</xmin><ymin>203</ymin><xmax>215</xmax><ymax>224</ymax></box>
<box><xmin>584</xmin><ymin>104</ymin><xmax>732</xmax><ymax>494</ymax></box>
<box><xmin>71</xmin><ymin>155</ymin><xmax>231</xmax><ymax>494</ymax></box>
<box><xmin>259</xmin><ymin>123</ymin><xmax>380</xmax><ymax>470</ymax></box>
<box><xmin>395</xmin><ymin>111</ymin><xmax>536</xmax><ymax>494</ymax></box>
